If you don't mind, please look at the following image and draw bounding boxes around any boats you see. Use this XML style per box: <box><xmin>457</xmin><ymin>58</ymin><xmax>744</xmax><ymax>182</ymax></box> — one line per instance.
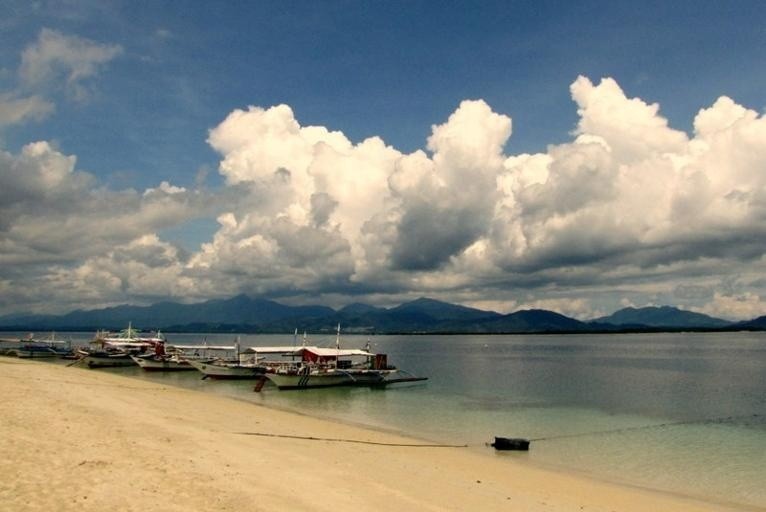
<box><xmin>261</xmin><ymin>323</ymin><xmax>429</xmax><ymax>392</ymax></box>
<box><xmin>183</xmin><ymin>327</ymin><xmax>319</xmax><ymax>380</ymax></box>
<box><xmin>0</xmin><ymin>320</ymin><xmax>235</xmax><ymax>372</ymax></box>
